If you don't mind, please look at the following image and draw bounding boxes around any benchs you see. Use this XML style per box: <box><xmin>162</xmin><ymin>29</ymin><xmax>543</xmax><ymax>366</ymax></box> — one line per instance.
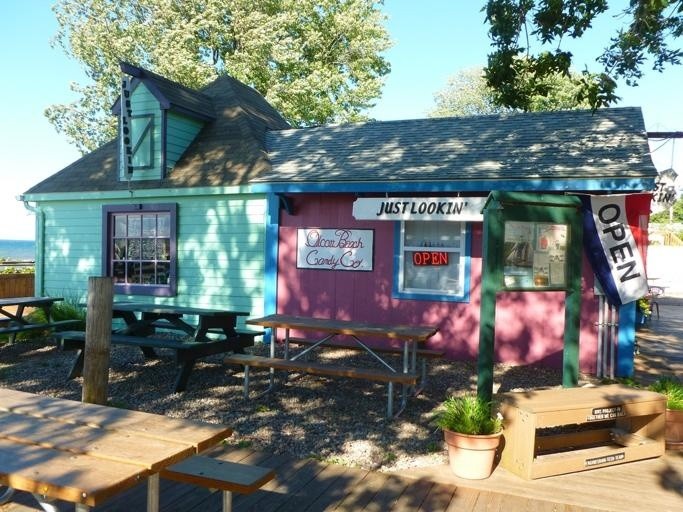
<box><xmin>161</xmin><ymin>455</ymin><xmax>276</xmax><ymax>512</ymax></box>
<box><xmin>489</xmin><ymin>383</ymin><xmax>668</xmax><ymax>480</ymax></box>
<box><xmin>0</xmin><ymin>499</ymin><xmax>43</xmax><ymax>512</ymax></box>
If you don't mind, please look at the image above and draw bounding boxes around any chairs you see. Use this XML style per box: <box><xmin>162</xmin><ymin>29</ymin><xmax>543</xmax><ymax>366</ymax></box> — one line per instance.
<box><xmin>649</xmin><ymin>286</ymin><xmax>665</xmax><ymax>320</ymax></box>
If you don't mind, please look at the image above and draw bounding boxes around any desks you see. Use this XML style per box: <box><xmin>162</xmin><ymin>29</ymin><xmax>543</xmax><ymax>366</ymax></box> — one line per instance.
<box><xmin>0</xmin><ymin>386</ymin><xmax>233</xmax><ymax>512</ymax></box>
<box><xmin>60</xmin><ymin>302</ymin><xmax>249</xmax><ymax>394</ymax></box>
<box><xmin>0</xmin><ymin>296</ymin><xmax>64</xmax><ymax>343</ymax></box>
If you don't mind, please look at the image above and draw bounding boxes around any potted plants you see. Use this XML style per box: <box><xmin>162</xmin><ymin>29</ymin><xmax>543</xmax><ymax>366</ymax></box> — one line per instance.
<box><xmin>649</xmin><ymin>373</ymin><xmax>683</xmax><ymax>450</ymax></box>
<box><xmin>431</xmin><ymin>393</ymin><xmax>504</xmax><ymax>480</ymax></box>
